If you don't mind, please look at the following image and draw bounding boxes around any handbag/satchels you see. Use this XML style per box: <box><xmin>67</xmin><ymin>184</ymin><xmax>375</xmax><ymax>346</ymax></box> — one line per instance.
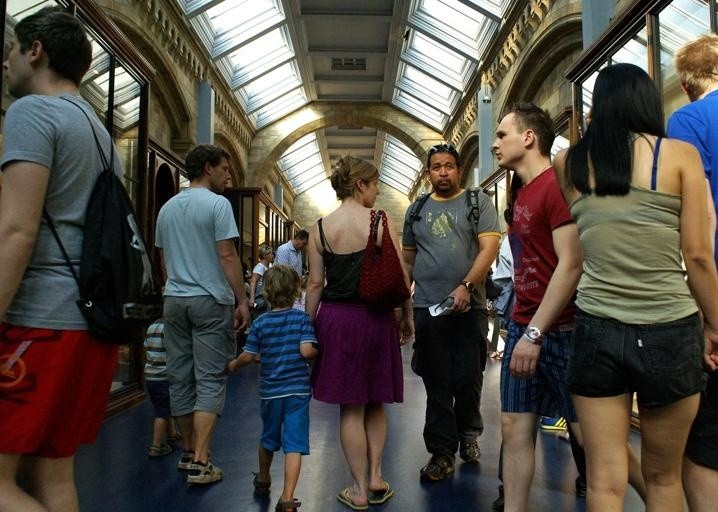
<box><xmin>360</xmin><ymin>211</ymin><xmax>410</xmax><ymax>310</ymax></box>
<box><xmin>245</xmin><ymin>286</ymin><xmax>265</xmax><ymax>310</ymax></box>
<box><xmin>486</xmin><ymin>275</ymin><xmax>503</xmax><ymax>301</ymax></box>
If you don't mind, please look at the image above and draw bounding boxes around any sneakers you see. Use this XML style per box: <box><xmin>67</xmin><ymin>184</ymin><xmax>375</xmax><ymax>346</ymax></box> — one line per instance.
<box><xmin>493</xmin><ymin>485</ymin><xmax>504</xmax><ymax>508</ymax></box>
<box><xmin>576</xmin><ymin>476</ymin><xmax>587</xmax><ymax>498</ymax></box>
<box><xmin>459</xmin><ymin>441</ymin><xmax>481</xmax><ymax>461</ymax></box>
<box><xmin>186</xmin><ymin>461</ymin><xmax>223</xmax><ymax>484</ymax></box>
<box><xmin>541</xmin><ymin>416</ymin><xmax>568</xmax><ymax>430</ymax></box>
<box><xmin>178</xmin><ymin>448</ymin><xmax>211</xmax><ymax>468</ymax></box>
<box><xmin>420</xmin><ymin>455</ymin><xmax>455</xmax><ymax>481</ymax></box>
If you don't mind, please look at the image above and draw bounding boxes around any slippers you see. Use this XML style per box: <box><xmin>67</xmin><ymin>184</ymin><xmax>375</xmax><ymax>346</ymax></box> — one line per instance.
<box><xmin>337</xmin><ymin>486</ymin><xmax>369</xmax><ymax>510</ymax></box>
<box><xmin>368</xmin><ymin>481</ymin><xmax>394</xmax><ymax>504</ymax></box>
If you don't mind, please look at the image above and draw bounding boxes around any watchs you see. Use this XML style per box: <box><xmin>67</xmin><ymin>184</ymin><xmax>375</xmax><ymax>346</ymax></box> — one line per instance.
<box><xmin>459</xmin><ymin>280</ymin><xmax>476</xmax><ymax>292</ymax></box>
<box><xmin>525</xmin><ymin>327</ymin><xmax>545</xmax><ymax>345</ymax></box>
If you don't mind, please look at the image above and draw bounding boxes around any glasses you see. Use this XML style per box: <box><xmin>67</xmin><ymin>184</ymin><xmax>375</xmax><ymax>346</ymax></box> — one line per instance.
<box><xmin>429</xmin><ymin>144</ymin><xmax>455</xmax><ymax>153</ymax></box>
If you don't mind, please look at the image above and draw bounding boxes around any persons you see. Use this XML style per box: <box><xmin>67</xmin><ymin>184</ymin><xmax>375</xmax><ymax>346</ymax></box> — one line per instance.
<box><xmin>248</xmin><ymin>229</ymin><xmax>309</xmax><ymax>362</ymax></box>
<box><xmin>306</xmin><ymin>153</ymin><xmax>416</xmax><ymax>510</ymax></box>
<box><xmin>667</xmin><ymin>36</ymin><xmax>718</xmax><ymax>511</ymax></box>
<box><xmin>488</xmin><ymin>237</ymin><xmax>517</xmax><ymax>359</ymax></box>
<box><xmin>0</xmin><ymin>11</ymin><xmax>163</xmax><ymax>512</ymax></box>
<box><xmin>145</xmin><ymin>145</ymin><xmax>249</xmax><ymax>483</ymax></box>
<box><xmin>554</xmin><ymin>63</ymin><xmax>718</xmax><ymax>512</ymax></box>
<box><xmin>230</xmin><ymin>264</ymin><xmax>319</xmax><ymax>507</ymax></box>
<box><xmin>402</xmin><ymin>146</ymin><xmax>501</xmax><ymax>479</ymax></box>
<box><xmin>494</xmin><ymin>105</ymin><xmax>648</xmax><ymax>511</ymax></box>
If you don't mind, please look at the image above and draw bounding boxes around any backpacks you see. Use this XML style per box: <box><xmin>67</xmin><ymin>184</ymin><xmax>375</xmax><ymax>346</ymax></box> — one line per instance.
<box><xmin>55</xmin><ymin>96</ymin><xmax>163</xmax><ymax>344</ymax></box>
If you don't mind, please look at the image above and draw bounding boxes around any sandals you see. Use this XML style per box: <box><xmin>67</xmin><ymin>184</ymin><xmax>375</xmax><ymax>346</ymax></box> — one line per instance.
<box><xmin>276</xmin><ymin>498</ymin><xmax>301</xmax><ymax>512</ymax></box>
<box><xmin>168</xmin><ymin>432</ymin><xmax>181</xmax><ymax>443</ymax></box>
<box><xmin>253</xmin><ymin>473</ymin><xmax>272</xmax><ymax>497</ymax></box>
<box><xmin>149</xmin><ymin>443</ymin><xmax>173</xmax><ymax>457</ymax></box>
